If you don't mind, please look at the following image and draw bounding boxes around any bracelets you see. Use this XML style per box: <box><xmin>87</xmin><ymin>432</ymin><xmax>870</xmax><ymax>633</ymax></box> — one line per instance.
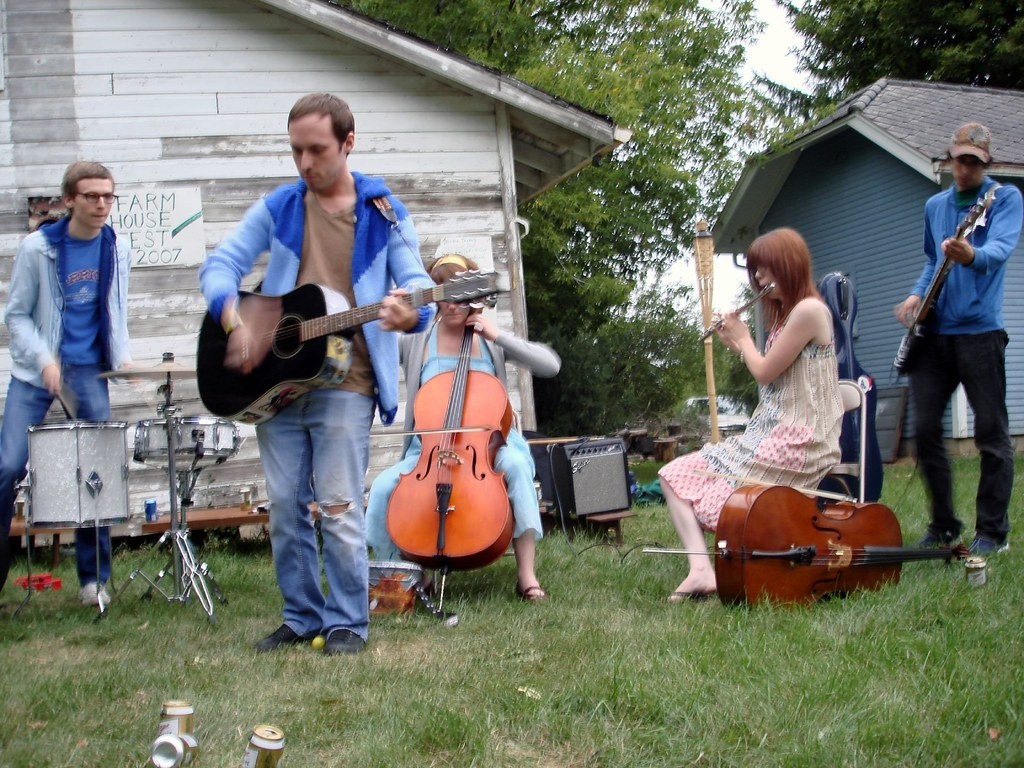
<box><xmin>225</xmin><ymin>322</ymin><xmax>241</xmax><ymax>340</ymax></box>
<box><xmin>740</xmin><ymin>350</ymin><xmax>744</xmax><ymax>364</ymax></box>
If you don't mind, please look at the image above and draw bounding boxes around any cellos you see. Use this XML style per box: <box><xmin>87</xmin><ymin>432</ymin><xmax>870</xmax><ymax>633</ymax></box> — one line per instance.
<box><xmin>640</xmin><ymin>483</ymin><xmax>971</xmax><ymax>611</ymax></box>
<box><xmin>384</xmin><ymin>287</ymin><xmax>515</xmax><ymax>617</ymax></box>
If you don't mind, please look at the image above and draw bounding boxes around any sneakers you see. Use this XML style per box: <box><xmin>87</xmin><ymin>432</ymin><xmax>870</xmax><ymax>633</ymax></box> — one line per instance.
<box><xmin>77</xmin><ymin>582</ymin><xmax>110</xmax><ymax>605</ymax></box>
<box><xmin>967</xmin><ymin>539</ymin><xmax>1010</xmax><ymax>559</ymax></box>
<box><xmin>323</xmin><ymin>629</ymin><xmax>364</xmax><ymax>656</ymax></box>
<box><xmin>253</xmin><ymin>624</ymin><xmax>302</xmax><ymax>652</ymax></box>
<box><xmin>917</xmin><ymin>534</ymin><xmax>962</xmax><ymax>549</ymax></box>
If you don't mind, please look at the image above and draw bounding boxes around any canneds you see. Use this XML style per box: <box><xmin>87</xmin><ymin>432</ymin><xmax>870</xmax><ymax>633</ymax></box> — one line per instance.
<box><xmin>238</xmin><ymin>724</ymin><xmax>285</xmax><ymax>768</ymax></box>
<box><xmin>144</xmin><ymin>498</ymin><xmax>159</xmax><ymax>522</ymax></box>
<box><xmin>238</xmin><ymin>488</ymin><xmax>251</xmax><ymax>511</ymax></box>
<box><xmin>964</xmin><ymin>556</ymin><xmax>987</xmax><ymax>587</ymax></box>
<box><xmin>149</xmin><ymin>734</ymin><xmax>201</xmax><ymax>768</ymax></box>
<box><xmin>158</xmin><ymin>701</ymin><xmax>194</xmax><ymax>736</ymax></box>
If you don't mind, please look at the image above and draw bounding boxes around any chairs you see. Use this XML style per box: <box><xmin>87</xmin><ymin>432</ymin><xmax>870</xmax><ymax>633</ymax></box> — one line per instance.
<box><xmin>827</xmin><ymin>380</ymin><xmax>868</xmax><ymax>505</ymax></box>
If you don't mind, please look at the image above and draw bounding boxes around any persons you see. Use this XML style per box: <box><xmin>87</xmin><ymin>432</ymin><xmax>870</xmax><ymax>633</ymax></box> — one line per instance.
<box><xmin>362</xmin><ymin>256</ymin><xmax>549</xmax><ymax>602</ymax></box>
<box><xmin>197</xmin><ymin>92</ymin><xmax>438</xmax><ymax>654</ymax></box>
<box><xmin>657</xmin><ymin>227</ymin><xmax>845</xmax><ymax>601</ymax></box>
<box><xmin>0</xmin><ymin>161</ymin><xmax>133</xmax><ymax>602</ymax></box>
<box><xmin>896</xmin><ymin>121</ymin><xmax>1024</xmax><ymax>557</ymax></box>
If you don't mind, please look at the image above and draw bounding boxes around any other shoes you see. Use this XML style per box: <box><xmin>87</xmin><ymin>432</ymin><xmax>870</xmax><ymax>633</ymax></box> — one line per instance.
<box><xmin>515</xmin><ymin>582</ymin><xmax>547</xmax><ymax>602</ymax></box>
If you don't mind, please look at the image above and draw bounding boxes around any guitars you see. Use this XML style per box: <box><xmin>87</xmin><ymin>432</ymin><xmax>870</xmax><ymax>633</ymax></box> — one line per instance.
<box><xmin>195</xmin><ymin>267</ymin><xmax>513</xmax><ymax>428</ymax></box>
<box><xmin>894</xmin><ymin>188</ymin><xmax>996</xmax><ymax>378</ymax></box>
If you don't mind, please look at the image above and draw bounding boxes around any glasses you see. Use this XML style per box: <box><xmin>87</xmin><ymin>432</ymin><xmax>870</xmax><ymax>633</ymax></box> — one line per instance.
<box><xmin>75</xmin><ymin>192</ymin><xmax>118</xmax><ymax>204</ymax></box>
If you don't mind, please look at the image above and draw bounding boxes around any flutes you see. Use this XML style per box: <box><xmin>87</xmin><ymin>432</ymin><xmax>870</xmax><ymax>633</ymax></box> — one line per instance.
<box><xmin>697</xmin><ymin>281</ymin><xmax>776</xmax><ymax>343</ymax></box>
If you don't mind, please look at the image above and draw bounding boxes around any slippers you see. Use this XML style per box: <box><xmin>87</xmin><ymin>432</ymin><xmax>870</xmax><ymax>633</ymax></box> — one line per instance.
<box><xmin>667</xmin><ymin>592</ymin><xmax>709</xmax><ymax>604</ymax></box>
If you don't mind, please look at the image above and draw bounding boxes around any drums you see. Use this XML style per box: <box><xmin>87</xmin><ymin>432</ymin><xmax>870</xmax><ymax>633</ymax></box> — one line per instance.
<box><xmin>26</xmin><ymin>419</ymin><xmax>132</xmax><ymax>531</ymax></box>
<box><xmin>134</xmin><ymin>415</ymin><xmax>238</xmax><ymax>465</ymax></box>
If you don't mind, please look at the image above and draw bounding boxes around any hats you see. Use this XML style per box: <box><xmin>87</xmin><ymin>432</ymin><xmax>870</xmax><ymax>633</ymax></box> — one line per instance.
<box><xmin>949</xmin><ymin>123</ymin><xmax>992</xmax><ymax>163</ymax></box>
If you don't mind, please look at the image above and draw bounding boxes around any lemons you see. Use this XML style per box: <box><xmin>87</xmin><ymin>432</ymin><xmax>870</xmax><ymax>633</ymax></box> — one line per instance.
<box><xmin>312</xmin><ymin>637</ymin><xmax>325</xmax><ymax>650</ymax></box>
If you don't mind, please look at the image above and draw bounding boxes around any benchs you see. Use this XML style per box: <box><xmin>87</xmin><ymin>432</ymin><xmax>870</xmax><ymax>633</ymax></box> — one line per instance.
<box><xmin>141</xmin><ymin>503</ymin><xmax>319</xmax><ymax>533</ymax></box>
<box><xmin>10</xmin><ymin>515</ymin><xmax>75</xmax><ymax>568</ymax></box>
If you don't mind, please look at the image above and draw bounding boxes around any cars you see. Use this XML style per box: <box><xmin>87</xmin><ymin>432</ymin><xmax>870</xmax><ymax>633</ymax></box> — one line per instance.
<box><xmin>672</xmin><ymin>394</ymin><xmax>751</xmax><ymax>444</ymax></box>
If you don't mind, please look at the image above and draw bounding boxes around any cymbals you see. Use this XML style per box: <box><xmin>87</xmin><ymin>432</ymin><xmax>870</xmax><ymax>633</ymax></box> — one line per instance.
<box><xmin>97</xmin><ymin>362</ymin><xmax>199</xmax><ymax>380</ymax></box>
